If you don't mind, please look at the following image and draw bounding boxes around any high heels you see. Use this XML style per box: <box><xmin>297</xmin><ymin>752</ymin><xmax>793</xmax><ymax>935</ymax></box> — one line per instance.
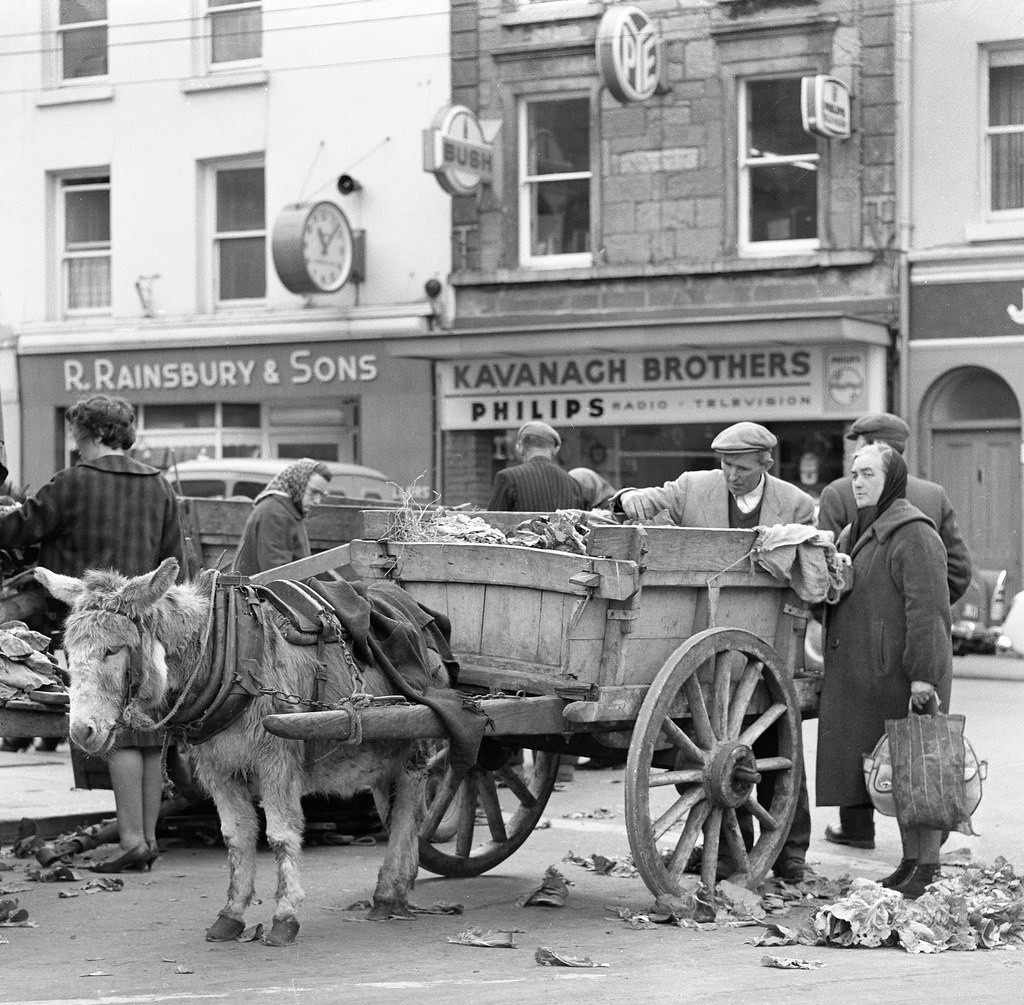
<box><xmin>92</xmin><ymin>840</ymin><xmax>159</xmax><ymax>872</ymax></box>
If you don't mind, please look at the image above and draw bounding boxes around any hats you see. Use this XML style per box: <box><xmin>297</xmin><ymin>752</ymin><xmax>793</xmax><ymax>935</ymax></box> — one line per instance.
<box><xmin>517</xmin><ymin>420</ymin><xmax>561</xmax><ymax>448</ymax></box>
<box><xmin>845</xmin><ymin>412</ymin><xmax>911</xmax><ymax>442</ymax></box>
<box><xmin>711</xmin><ymin>423</ymin><xmax>778</xmax><ymax>454</ymax></box>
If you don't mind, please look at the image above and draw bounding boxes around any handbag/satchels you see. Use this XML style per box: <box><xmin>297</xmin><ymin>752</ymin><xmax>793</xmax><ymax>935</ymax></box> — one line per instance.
<box><xmin>884</xmin><ymin>690</ymin><xmax>982</xmax><ymax>837</ymax></box>
<box><xmin>861</xmin><ymin>691</ymin><xmax>988</xmax><ymax>819</ymax></box>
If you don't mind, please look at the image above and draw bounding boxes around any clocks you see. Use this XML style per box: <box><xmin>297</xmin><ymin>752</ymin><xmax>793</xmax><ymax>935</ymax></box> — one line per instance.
<box><xmin>273</xmin><ymin>199</ymin><xmax>366</xmax><ymax>296</ymax></box>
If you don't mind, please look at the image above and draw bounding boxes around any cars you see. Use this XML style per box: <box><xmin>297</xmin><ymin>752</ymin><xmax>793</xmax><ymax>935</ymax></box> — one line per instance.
<box><xmin>166</xmin><ymin>457</ymin><xmax>392</xmax><ymax>516</ymax></box>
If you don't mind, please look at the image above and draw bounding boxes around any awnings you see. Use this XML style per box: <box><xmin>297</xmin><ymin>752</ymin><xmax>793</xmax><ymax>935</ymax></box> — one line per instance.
<box><xmin>376</xmin><ymin>309</ymin><xmax>894</xmax><ymax>362</ymax></box>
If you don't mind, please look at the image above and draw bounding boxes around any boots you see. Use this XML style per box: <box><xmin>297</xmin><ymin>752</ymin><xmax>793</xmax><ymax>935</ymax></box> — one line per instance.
<box><xmin>874</xmin><ymin>857</ymin><xmax>943</xmax><ymax>900</ymax></box>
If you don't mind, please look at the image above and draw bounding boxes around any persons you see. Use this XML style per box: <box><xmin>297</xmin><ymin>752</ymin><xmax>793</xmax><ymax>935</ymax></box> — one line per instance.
<box><xmin>805</xmin><ymin>409</ymin><xmax>974</xmax><ymax>846</ymax></box>
<box><xmin>609</xmin><ymin>418</ymin><xmax>824</xmax><ymax>887</ymax></box>
<box><xmin>0</xmin><ymin>393</ymin><xmax>184</xmax><ymax>874</ymax></box>
<box><xmin>816</xmin><ymin>438</ymin><xmax>956</xmax><ymax>901</ymax></box>
<box><xmin>483</xmin><ymin>418</ymin><xmax>590</xmax><ymax>793</ymax></box>
<box><xmin>566</xmin><ymin>468</ymin><xmax>617</xmax><ymax>513</ymax></box>
<box><xmin>232</xmin><ymin>455</ymin><xmax>332</xmax><ymax>578</ymax></box>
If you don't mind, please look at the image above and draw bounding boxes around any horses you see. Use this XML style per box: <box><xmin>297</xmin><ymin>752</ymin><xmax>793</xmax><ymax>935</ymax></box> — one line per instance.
<box><xmin>33</xmin><ymin>553</ymin><xmax>455</xmax><ymax>946</ymax></box>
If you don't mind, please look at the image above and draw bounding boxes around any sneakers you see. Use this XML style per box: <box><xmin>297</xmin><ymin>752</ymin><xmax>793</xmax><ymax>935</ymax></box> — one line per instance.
<box><xmin>825</xmin><ymin>824</ymin><xmax>876</xmax><ymax>849</ymax></box>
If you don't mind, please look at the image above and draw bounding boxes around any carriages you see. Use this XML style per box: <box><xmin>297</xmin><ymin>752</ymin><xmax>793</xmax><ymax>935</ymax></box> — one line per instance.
<box><xmin>36</xmin><ymin>514</ymin><xmax>831</xmax><ymax>943</ymax></box>
